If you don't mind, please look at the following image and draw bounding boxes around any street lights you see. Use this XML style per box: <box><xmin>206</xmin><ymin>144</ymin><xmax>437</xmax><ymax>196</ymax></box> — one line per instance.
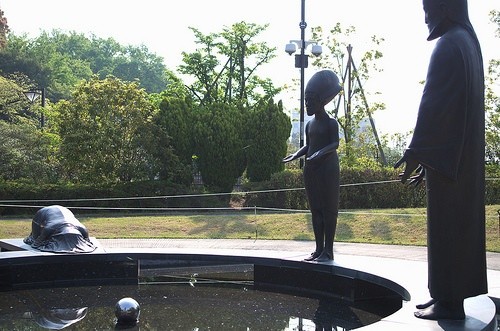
<box><xmin>22</xmin><ymin>88</ymin><xmax>46</xmax><ymax>128</ymax></box>
<box><xmin>284</xmin><ymin>0</ymin><xmax>323</xmax><ymax>169</ymax></box>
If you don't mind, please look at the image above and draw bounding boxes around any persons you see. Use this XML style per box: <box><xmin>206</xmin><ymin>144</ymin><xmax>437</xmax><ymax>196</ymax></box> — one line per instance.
<box><xmin>24</xmin><ymin>205</ymin><xmax>98</xmax><ymax>254</ymax></box>
<box><xmin>394</xmin><ymin>0</ymin><xmax>489</xmax><ymax>321</ymax></box>
<box><xmin>282</xmin><ymin>70</ymin><xmax>343</xmax><ymax>262</ymax></box>
